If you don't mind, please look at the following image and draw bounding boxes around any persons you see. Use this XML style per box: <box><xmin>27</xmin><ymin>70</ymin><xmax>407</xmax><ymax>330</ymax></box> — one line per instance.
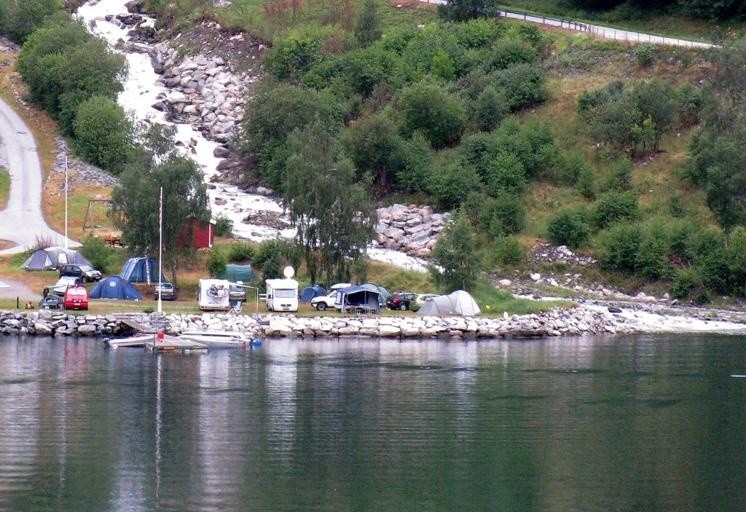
<box><xmin>42</xmin><ymin>287</ymin><xmax>49</xmax><ymax>299</ymax></box>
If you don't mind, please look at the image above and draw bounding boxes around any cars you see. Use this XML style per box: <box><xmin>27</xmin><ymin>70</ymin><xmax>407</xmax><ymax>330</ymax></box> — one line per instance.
<box><xmin>154</xmin><ymin>282</ymin><xmax>178</xmax><ymax>301</ymax></box>
<box><xmin>386</xmin><ymin>291</ymin><xmax>442</xmax><ymax>311</ymax></box>
<box><xmin>63</xmin><ymin>286</ymin><xmax>89</xmax><ymax>310</ymax></box>
<box><xmin>228</xmin><ymin>285</ymin><xmax>247</xmax><ymax>302</ymax></box>
<box><xmin>310</xmin><ymin>288</ymin><xmax>339</xmax><ymax>312</ymax></box>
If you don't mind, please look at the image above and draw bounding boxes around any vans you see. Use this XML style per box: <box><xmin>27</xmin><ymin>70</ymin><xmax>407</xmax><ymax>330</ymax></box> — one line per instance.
<box><xmin>58</xmin><ymin>263</ymin><xmax>103</xmax><ymax>284</ymax></box>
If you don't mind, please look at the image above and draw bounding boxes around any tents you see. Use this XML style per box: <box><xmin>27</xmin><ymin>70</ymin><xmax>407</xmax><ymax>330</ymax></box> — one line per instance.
<box><xmin>222</xmin><ymin>264</ymin><xmax>259</xmax><ymax>282</ymax></box>
<box><xmin>120</xmin><ymin>256</ymin><xmax>168</xmax><ymax>283</ymax></box>
<box><xmin>300</xmin><ymin>284</ymin><xmax>328</xmax><ymax>302</ymax></box>
<box><xmin>333</xmin><ymin>285</ymin><xmax>382</xmax><ymax>312</ymax></box>
<box><xmin>20</xmin><ymin>246</ymin><xmax>94</xmax><ymax>271</ymax></box>
<box><xmin>416</xmin><ymin>289</ymin><xmax>483</xmax><ymax>318</ymax></box>
<box><xmin>378</xmin><ymin>287</ymin><xmax>391</xmax><ymax>306</ymax></box>
<box><xmin>89</xmin><ymin>274</ymin><xmax>142</xmax><ymax>301</ymax></box>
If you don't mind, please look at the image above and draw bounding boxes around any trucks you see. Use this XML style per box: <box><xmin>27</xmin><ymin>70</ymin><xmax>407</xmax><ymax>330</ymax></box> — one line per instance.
<box><xmin>265</xmin><ymin>278</ymin><xmax>299</xmax><ymax>312</ymax></box>
